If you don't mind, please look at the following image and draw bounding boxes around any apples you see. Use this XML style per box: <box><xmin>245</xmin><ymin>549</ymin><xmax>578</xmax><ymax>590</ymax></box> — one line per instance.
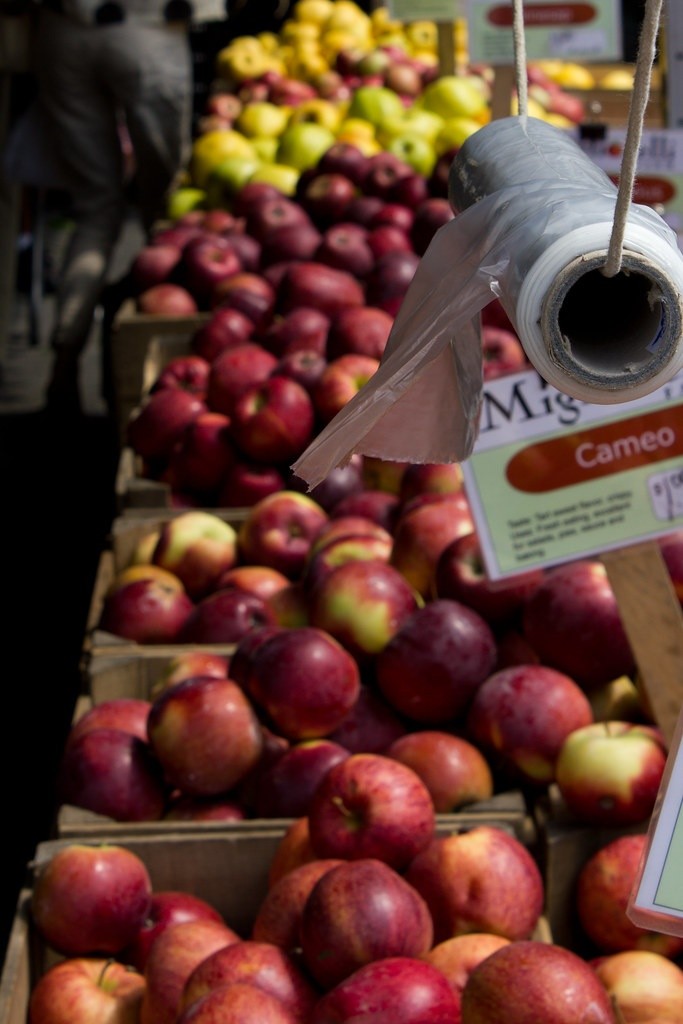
<box><xmin>32</xmin><ymin>0</ymin><xmax>683</xmax><ymax>1024</ymax></box>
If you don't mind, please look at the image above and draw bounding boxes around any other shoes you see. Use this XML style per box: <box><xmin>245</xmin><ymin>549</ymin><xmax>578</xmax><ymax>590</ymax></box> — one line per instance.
<box><xmin>49</xmin><ymin>249</ymin><xmax>102</xmax><ymax>357</ymax></box>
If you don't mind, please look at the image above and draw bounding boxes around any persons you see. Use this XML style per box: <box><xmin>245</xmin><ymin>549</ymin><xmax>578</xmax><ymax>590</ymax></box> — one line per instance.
<box><xmin>14</xmin><ymin>0</ymin><xmax>215</xmax><ymax>415</ymax></box>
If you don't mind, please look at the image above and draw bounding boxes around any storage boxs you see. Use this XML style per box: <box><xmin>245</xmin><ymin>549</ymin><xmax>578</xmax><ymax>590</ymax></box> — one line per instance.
<box><xmin>0</xmin><ymin>301</ymin><xmax>555</xmax><ymax>1024</ymax></box>
